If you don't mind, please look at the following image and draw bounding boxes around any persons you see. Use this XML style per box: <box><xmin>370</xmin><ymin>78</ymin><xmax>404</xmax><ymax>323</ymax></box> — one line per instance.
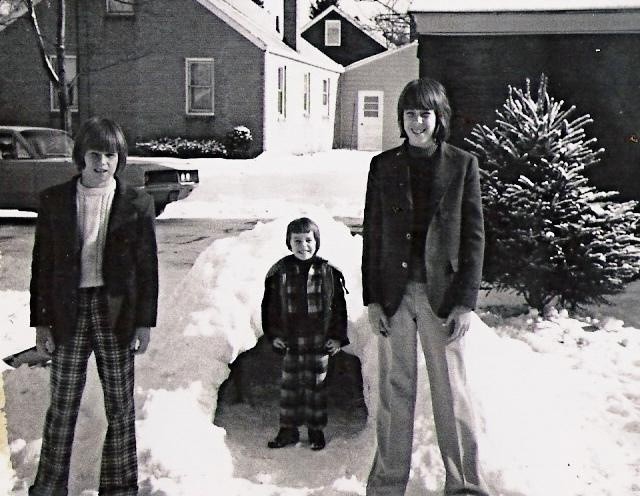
<box><xmin>27</xmin><ymin>114</ymin><xmax>161</xmax><ymax>496</ymax></box>
<box><xmin>360</xmin><ymin>77</ymin><xmax>494</xmax><ymax>496</ymax></box>
<box><xmin>258</xmin><ymin>215</ymin><xmax>350</xmax><ymax>451</ymax></box>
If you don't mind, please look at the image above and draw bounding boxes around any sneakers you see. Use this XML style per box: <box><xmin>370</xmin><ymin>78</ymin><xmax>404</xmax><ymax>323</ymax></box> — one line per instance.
<box><xmin>268</xmin><ymin>427</ymin><xmax>299</xmax><ymax>448</ymax></box>
<box><xmin>308</xmin><ymin>427</ymin><xmax>325</xmax><ymax>450</ymax></box>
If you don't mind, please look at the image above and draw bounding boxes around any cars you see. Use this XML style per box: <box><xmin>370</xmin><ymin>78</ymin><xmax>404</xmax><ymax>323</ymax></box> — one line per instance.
<box><xmin>1</xmin><ymin>126</ymin><xmax>200</xmax><ymax>220</ymax></box>
